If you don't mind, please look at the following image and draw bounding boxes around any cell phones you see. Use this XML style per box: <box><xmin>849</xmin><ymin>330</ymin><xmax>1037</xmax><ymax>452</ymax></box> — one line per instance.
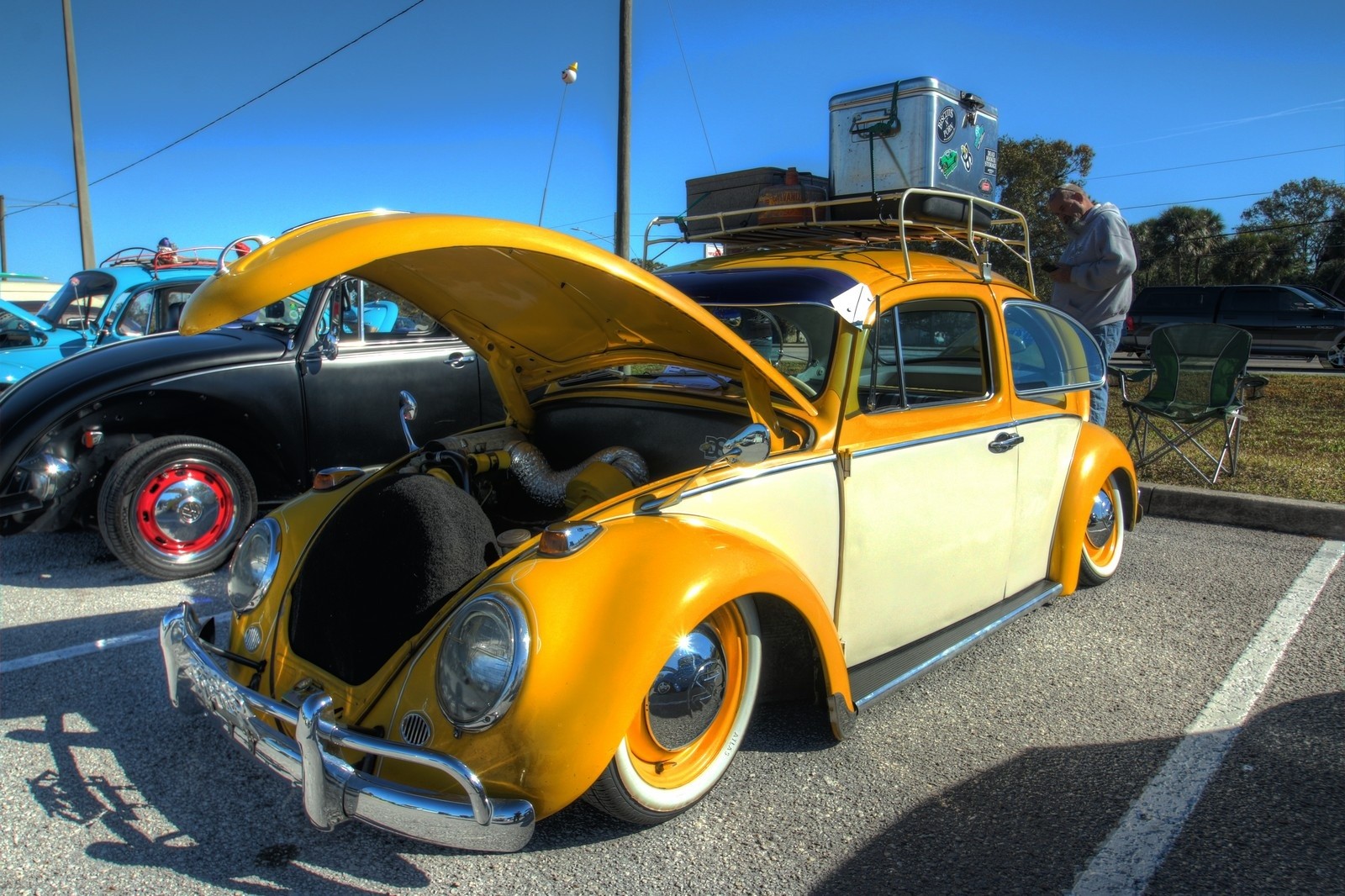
<box><xmin>1040</xmin><ymin>262</ymin><xmax>1059</xmax><ymax>272</ymax></box>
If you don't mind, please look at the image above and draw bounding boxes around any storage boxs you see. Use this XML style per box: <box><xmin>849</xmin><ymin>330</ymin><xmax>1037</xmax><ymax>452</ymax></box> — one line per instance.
<box><xmin>685</xmin><ymin>167</ymin><xmax>829</xmax><ymax>234</ymax></box>
<box><xmin>826</xmin><ymin>77</ymin><xmax>999</xmax><ymax>232</ymax></box>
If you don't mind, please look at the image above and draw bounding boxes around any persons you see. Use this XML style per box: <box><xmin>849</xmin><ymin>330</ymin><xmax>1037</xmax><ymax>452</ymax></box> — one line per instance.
<box><xmin>157</xmin><ymin>238</ymin><xmax>201</xmax><ymax>332</ymax></box>
<box><xmin>1042</xmin><ymin>183</ymin><xmax>1136</xmax><ymax>432</ymax></box>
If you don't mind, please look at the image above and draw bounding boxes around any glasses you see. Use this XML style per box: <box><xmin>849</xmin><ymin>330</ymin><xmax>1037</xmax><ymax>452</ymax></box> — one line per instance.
<box><xmin>1049</xmin><ymin>188</ymin><xmax>1075</xmax><ymax>202</ymax></box>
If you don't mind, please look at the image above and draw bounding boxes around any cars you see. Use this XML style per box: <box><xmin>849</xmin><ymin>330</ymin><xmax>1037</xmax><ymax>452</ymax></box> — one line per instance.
<box><xmin>0</xmin><ymin>246</ymin><xmax>623</xmax><ymax>582</ymax></box>
<box><xmin>158</xmin><ymin>184</ymin><xmax>1143</xmax><ymax>855</ymax></box>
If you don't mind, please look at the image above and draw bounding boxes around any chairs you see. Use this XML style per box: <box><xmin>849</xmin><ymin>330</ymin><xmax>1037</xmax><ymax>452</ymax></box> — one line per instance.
<box><xmin>1106</xmin><ymin>321</ymin><xmax>1271</xmax><ymax>488</ymax></box>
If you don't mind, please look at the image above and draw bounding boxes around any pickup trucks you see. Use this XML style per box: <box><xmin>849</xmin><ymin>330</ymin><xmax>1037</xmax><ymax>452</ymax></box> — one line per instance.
<box><xmin>1118</xmin><ymin>285</ymin><xmax>1344</xmax><ymax>372</ymax></box>
